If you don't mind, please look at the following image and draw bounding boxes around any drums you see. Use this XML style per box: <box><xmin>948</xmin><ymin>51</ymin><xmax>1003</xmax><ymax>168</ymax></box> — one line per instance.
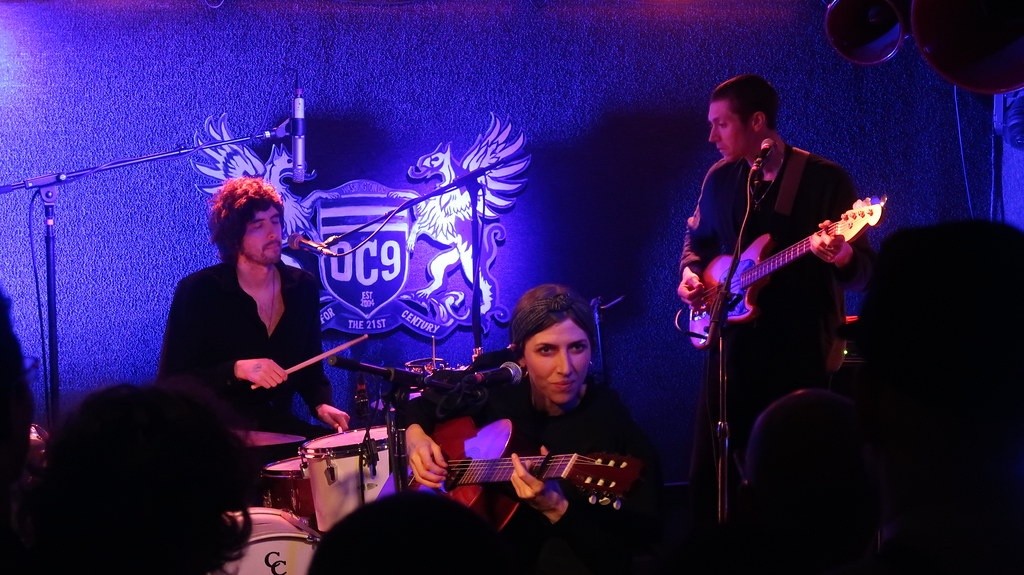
<box><xmin>298</xmin><ymin>424</ymin><xmax>401</xmax><ymax>534</ymax></box>
<box><xmin>260</xmin><ymin>455</ymin><xmax>318</xmax><ymax>529</ymax></box>
<box><xmin>208</xmin><ymin>505</ymin><xmax>324</xmax><ymax>575</ymax></box>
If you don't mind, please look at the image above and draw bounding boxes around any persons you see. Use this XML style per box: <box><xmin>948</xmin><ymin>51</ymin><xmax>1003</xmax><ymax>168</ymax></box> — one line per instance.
<box><xmin>157</xmin><ymin>177</ymin><xmax>352</xmax><ymax>465</ymax></box>
<box><xmin>0</xmin><ymin>219</ymin><xmax>1024</xmax><ymax>575</ymax></box>
<box><xmin>397</xmin><ymin>284</ymin><xmax>649</xmax><ymax>574</ymax></box>
<box><xmin>677</xmin><ymin>75</ymin><xmax>878</xmax><ymax>493</ymax></box>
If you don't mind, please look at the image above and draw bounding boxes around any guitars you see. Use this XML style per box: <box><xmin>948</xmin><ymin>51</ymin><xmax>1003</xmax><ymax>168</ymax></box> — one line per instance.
<box><xmin>688</xmin><ymin>193</ymin><xmax>893</xmax><ymax>352</ymax></box>
<box><xmin>375</xmin><ymin>415</ymin><xmax>651</xmax><ymax>531</ymax></box>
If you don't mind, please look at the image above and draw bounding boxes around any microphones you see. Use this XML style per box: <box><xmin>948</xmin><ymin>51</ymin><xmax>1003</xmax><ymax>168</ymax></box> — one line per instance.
<box><xmin>751</xmin><ymin>138</ymin><xmax>777</xmax><ymax>173</ymax></box>
<box><xmin>286</xmin><ymin>233</ymin><xmax>338</xmax><ymax>257</ymax></box>
<box><xmin>463</xmin><ymin>362</ymin><xmax>521</xmax><ymax>385</ymax></box>
<box><xmin>291</xmin><ymin>70</ymin><xmax>306</xmax><ymax>184</ymax></box>
